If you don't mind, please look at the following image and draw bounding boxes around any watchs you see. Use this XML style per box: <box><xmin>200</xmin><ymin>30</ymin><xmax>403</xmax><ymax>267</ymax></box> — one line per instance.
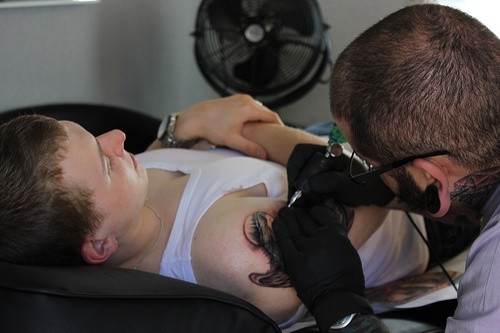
<box><xmin>329</xmin><ymin>313</ymin><xmax>358</xmax><ymax>329</ymax></box>
<box><xmin>157</xmin><ymin>112</ymin><xmax>184</xmax><ymax>148</ymax></box>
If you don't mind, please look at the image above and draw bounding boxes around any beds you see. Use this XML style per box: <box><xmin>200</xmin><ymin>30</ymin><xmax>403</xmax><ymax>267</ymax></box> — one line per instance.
<box><xmin>282</xmin><ymin>247</ymin><xmax>473</xmax><ymax>333</ymax></box>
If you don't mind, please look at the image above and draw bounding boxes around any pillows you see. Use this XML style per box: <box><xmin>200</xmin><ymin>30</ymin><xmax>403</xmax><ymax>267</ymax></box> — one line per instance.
<box><xmin>0</xmin><ymin>260</ymin><xmax>282</xmax><ymax>333</ymax></box>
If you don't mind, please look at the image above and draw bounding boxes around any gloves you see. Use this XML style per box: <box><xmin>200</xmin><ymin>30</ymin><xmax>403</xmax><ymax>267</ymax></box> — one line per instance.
<box><xmin>272</xmin><ymin>142</ymin><xmax>397</xmax><ymax>331</ymax></box>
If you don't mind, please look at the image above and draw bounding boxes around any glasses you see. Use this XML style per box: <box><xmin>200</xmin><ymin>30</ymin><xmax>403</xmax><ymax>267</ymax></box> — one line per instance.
<box><xmin>349</xmin><ymin>146</ymin><xmax>452</xmax><ymax>186</ymax></box>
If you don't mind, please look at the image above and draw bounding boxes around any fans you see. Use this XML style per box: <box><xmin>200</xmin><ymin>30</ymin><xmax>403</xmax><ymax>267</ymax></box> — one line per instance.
<box><xmin>190</xmin><ymin>0</ymin><xmax>334</xmax><ymax>108</ymax></box>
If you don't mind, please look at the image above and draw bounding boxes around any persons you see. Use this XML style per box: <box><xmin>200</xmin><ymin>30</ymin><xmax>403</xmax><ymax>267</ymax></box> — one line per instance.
<box><xmin>271</xmin><ymin>4</ymin><xmax>500</xmax><ymax>333</ymax></box>
<box><xmin>0</xmin><ymin>94</ymin><xmax>484</xmax><ymax>331</ymax></box>
